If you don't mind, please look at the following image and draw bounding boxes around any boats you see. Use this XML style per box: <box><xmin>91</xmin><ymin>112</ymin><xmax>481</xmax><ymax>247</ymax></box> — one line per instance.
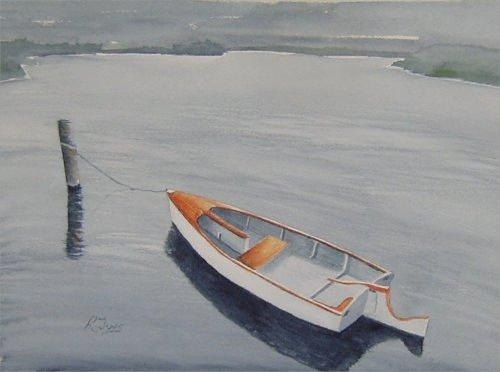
<box><xmin>165</xmin><ymin>187</ymin><xmax>430</xmax><ymax>339</ymax></box>
